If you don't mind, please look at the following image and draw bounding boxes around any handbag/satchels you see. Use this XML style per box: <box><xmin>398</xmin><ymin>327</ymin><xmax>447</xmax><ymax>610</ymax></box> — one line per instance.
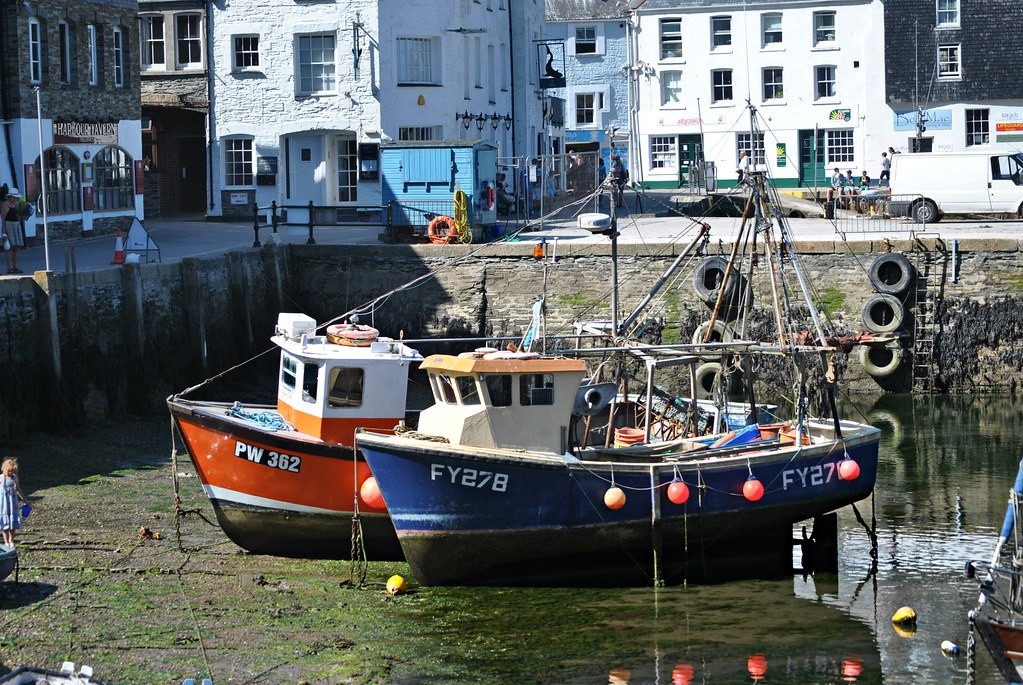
<box><xmin>2</xmin><ymin>233</ymin><xmax>10</xmax><ymax>250</ymax></box>
<box><xmin>619</xmin><ymin>163</ymin><xmax>629</xmax><ymax>179</ymax></box>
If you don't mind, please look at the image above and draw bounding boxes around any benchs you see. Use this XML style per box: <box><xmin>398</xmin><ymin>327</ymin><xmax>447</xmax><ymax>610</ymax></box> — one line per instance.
<box><xmin>805</xmin><ymin>421</ymin><xmax>863</xmax><ymax>439</ymax></box>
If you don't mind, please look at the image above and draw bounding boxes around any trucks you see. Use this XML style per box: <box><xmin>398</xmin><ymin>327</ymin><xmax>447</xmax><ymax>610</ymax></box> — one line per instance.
<box><xmin>887</xmin><ymin>144</ymin><xmax>1023</xmax><ymax>225</ymax></box>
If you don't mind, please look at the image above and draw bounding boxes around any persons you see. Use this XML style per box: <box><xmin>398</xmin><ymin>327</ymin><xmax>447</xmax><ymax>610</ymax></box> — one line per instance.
<box><xmin>827</xmin><ymin>168</ymin><xmax>846</xmax><ymax>203</ymax></box>
<box><xmin>859</xmin><ymin>171</ymin><xmax>870</xmax><ymax>190</ymax></box>
<box><xmin>0</xmin><ymin>187</ymin><xmax>24</xmax><ymax>274</ymax></box>
<box><xmin>878</xmin><ymin>153</ymin><xmax>889</xmax><ymax>187</ymax></box>
<box><xmin>496</xmin><ymin>150</ymin><xmax>628</xmax><ymax>214</ymax></box>
<box><xmin>0</xmin><ymin>459</ymin><xmax>28</xmax><ymax>548</ymax></box>
<box><xmin>889</xmin><ymin>147</ymin><xmax>895</xmax><ymax>168</ymax></box>
<box><xmin>845</xmin><ymin>170</ymin><xmax>855</xmax><ymax>205</ymax></box>
<box><xmin>737</xmin><ymin>150</ymin><xmax>749</xmax><ymax>192</ymax></box>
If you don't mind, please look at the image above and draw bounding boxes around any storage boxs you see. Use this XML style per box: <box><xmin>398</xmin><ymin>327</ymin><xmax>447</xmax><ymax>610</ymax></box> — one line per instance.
<box><xmin>277</xmin><ymin>312</ymin><xmax>316</xmax><ymax>339</ymax></box>
<box><xmin>721</xmin><ymin>423</ymin><xmax>759</xmax><ymax>447</ymax></box>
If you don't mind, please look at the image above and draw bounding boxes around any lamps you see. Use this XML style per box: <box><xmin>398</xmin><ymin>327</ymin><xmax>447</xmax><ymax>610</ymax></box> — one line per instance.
<box><xmin>455</xmin><ymin>111</ymin><xmax>514</xmax><ymax>132</ymax></box>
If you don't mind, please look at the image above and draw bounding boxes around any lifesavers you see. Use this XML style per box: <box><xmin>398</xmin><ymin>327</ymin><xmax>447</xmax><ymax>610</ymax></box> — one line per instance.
<box><xmin>869</xmin><ymin>252</ymin><xmax>912</xmax><ymax>294</ymax></box>
<box><xmin>428</xmin><ymin>216</ymin><xmax>456</xmax><ymax>244</ymax></box>
<box><xmin>692</xmin><ymin>256</ymin><xmax>737</xmax><ymax>303</ymax></box>
<box><xmin>326</xmin><ymin>324</ymin><xmax>380</xmax><ymax>347</ymax></box>
<box><xmin>692</xmin><ymin>320</ymin><xmax>734</xmax><ymax>362</ymax></box>
<box><xmin>862</xmin><ymin>409</ymin><xmax>905</xmax><ymax>454</ymax></box>
<box><xmin>861</xmin><ymin>293</ymin><xmax>905</xmax><ymax>334</ymax></box>
<box><xmin>695</xmin><ymin>362</ymin><xmax>732</xmax><ymax>399</ymax></box>
<box><xmin>486</xmin><ymin>187</ymin><xmax>495</xmax><ymax>211</ymax></box>
<box><xmin>860</xmin><ymin>340</ymin><xmax>903</xmax><ymax>377</ymax></box>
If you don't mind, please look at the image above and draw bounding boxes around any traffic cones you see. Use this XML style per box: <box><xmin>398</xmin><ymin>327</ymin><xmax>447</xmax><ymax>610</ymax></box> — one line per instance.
<box><xmin>110</xmin><ymin>227</ymin><xmax>126</xmax><ymax>264</ymax></box>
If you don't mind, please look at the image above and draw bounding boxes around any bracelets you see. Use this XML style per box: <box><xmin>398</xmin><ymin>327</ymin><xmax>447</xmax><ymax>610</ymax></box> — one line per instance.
<box><xmin>3</xmin><ymin>234</ymin><xmax>7</xmax><ymax>237</ymax></box>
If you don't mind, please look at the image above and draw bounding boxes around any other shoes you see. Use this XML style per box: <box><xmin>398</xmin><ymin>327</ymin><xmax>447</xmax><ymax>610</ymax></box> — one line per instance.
<box><xmin>5</xmin><ymin>543</ymin><xmax>16</xmax><ymax>550</ymax></box>
<box><xmin>887</xmin><ymin>182</ymin><xmax>889</xmax><ymax>187</ymax></box>
<box><xmin>7</xmin><ymin>269</ymin><xmax>23</xmax><ymax>273</ymax></box>
<box><xmin>875</xmin><ymin>184</ymin><xmax>881</xmax><ymax>188</ymax></box>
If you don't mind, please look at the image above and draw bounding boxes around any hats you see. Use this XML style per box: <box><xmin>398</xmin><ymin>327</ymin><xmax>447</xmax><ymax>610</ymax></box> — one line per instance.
<box><xmin>6</xmin><ymin>187</ymin><xmax>22</xmax><ymax>198</ymax></box>
<box><xmin>612</xmin><ymin>156</ymin><xmax>620</xmax><ymax>160</ymax></box>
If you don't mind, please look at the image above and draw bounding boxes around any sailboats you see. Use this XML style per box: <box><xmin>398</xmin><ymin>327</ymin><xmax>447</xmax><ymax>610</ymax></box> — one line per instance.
<box><xmin>164</xmin><ymin>91</ymin><xmax>889</xmax><ymax>590</ymax></box>
